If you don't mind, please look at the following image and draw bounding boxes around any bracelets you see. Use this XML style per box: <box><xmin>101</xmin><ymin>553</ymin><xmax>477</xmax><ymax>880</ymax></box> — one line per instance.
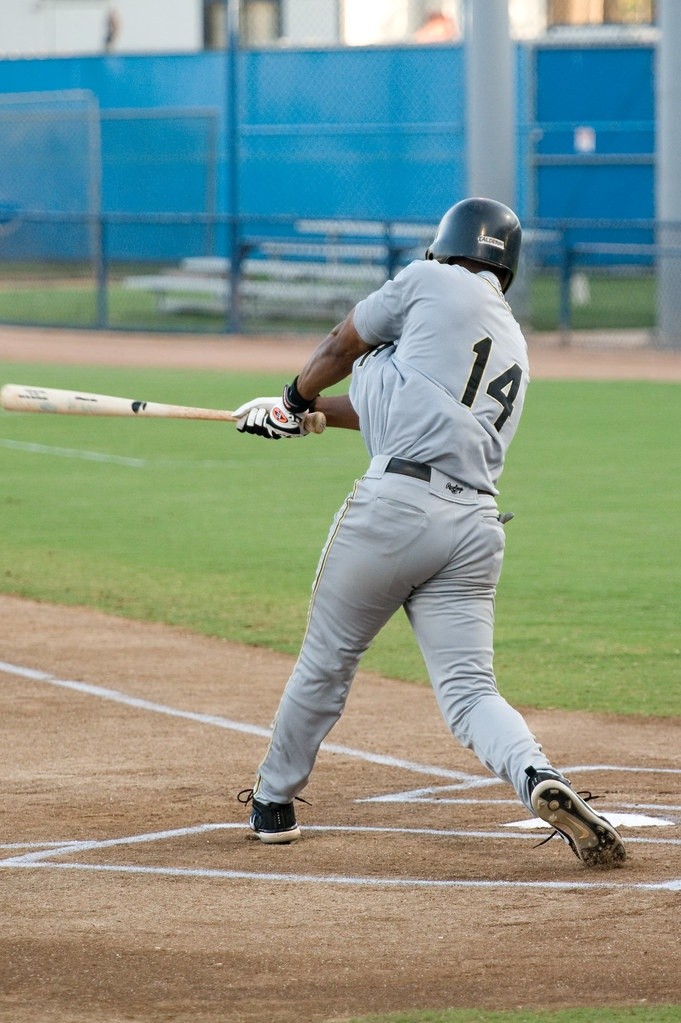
<box><xmin>289</xmin><ymin>375</ymin><xmax>320</xmax><ymax>407</ymax></box>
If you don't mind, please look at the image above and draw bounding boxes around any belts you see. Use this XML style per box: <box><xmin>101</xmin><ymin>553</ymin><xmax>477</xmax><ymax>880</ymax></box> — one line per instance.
<box><xmin>385</xmin><ymin>457</ymin><xmax>494</xmax><ymax>497</ymax></box>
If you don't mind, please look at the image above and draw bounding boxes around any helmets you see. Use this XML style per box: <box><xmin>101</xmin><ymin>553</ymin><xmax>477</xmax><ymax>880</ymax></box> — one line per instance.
<box><xmin>425</xmin><ymin>198</ymin><xmax>521</xmax><ymax>295</ymax></box>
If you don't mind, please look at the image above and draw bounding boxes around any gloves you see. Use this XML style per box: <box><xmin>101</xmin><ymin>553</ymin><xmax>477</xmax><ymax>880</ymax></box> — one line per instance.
<box><xmin>231</xmin><ymin>375</ymin><xmax>320</xmax><ymax>440</ymax></box>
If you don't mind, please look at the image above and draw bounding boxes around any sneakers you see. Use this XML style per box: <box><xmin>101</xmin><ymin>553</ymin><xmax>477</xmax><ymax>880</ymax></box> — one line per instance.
<box><xmin>237</xmin><ymin>789</ymin><xmax>312</xmax><ymax>844</ymax></box>
<box><xmin>524</xmin><ymin>765</ymin><xmax>628</xmax><ymax>871</ymax></box>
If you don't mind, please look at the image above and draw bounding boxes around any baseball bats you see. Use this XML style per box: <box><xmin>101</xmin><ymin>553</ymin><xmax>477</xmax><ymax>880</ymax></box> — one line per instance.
<box><xmin>0</xmin><ymin>382</ymin><xmax>327</xmax><ymax>435</ymax></box>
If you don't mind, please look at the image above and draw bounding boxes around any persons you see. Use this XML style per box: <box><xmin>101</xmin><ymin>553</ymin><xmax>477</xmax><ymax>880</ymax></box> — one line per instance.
<box><xmin>231</xmin><ymin>198</ymin><xmax>624</xmax><ymax>869</ymax></box>
<box><xmin>413</xmin><ymin>13</ymin><xmax>458</xmax><ymax>43</ymax></box>
